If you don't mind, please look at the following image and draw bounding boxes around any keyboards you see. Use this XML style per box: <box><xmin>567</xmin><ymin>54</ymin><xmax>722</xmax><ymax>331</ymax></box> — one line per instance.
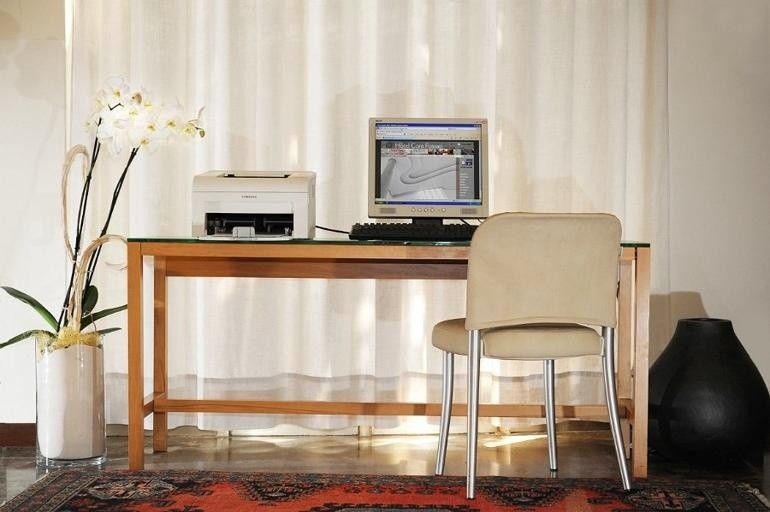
<box><xmin>349</xmin><ymin>222</ymin><xmax>479</xmax><ymax>241</ymax></box>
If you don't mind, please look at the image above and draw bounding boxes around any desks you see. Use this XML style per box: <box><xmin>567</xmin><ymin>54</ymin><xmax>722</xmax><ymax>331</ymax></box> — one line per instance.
<box><xmin>128</xmin><ymin>239</ymin><xmax>649</xmax><ymax>479</ymax></box>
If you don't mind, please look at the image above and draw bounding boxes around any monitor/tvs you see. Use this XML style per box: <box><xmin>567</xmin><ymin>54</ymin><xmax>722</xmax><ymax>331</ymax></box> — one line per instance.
<box><xmin>366</xmin><ymin>116</ymin><xmax>490</xmax><ymax>223</ymax></box>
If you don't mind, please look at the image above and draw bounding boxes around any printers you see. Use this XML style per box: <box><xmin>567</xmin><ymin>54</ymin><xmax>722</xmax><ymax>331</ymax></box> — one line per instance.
<box><xmin>190</xmin><ymin>169</ymin><xmax>317</xmax><ymax>241</ymax></box>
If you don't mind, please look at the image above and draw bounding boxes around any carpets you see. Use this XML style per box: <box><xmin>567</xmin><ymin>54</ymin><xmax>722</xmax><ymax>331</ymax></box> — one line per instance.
<box><xmin>0</xmin><ymin>471</ymin><xmax>770</xmax><ymax>512</ymax></box>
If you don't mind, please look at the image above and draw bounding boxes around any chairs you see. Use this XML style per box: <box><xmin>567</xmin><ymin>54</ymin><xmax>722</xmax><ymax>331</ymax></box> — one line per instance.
<box><xmin>434</xmin><ymin>212</ymin><xmax>631</xmax><ymax>500</ymax></box>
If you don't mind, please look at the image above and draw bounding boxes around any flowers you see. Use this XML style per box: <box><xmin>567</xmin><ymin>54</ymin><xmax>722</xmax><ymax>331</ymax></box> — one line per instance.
<box><xmin>0</xmin><ymin>84</ymin><xmax>205</xmax><ymax>363</ymax></box>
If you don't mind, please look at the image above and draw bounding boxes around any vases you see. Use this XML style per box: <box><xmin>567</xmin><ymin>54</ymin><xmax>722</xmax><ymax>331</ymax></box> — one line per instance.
<box><xmin>33</xmin><ymin>336</ymin><xmax>107</xmax><ymax>467</ymax></box>
<box><xmin>649</xmin><ymin>316</ymin><xmax>768</xmax><ymax>469</ymax></box>
